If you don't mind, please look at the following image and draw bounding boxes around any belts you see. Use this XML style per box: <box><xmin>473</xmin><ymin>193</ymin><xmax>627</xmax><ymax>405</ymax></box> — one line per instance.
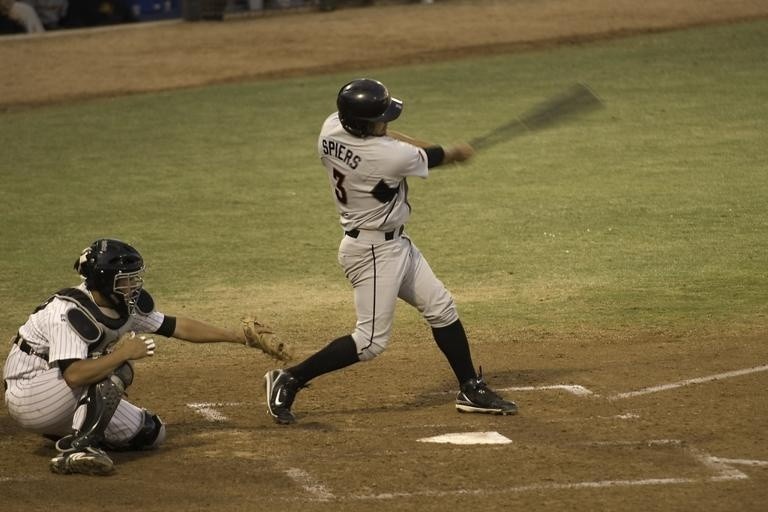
<box><xmin>347</xmin><ymin>224</ymin><xmax>404</xmax><ymax>241</ymax></box>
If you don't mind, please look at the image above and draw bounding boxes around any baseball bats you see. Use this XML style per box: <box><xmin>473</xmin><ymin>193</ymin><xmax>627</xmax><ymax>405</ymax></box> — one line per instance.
<box><xmin>464</xmin><ymin>85</ymin><xmax>605</xmax><ymax>152</ymax></box>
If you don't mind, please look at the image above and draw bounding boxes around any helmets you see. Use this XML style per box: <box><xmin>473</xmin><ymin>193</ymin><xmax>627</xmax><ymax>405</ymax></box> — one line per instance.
<box><xmin>337</xmin><ymin>78</ymin><xmax>403</xmax><ymax>137</ymax></box>
<box><xmin>75</xmin><ymin>238</ymin><xmax>145</xmax><ymax>319</ymax></box>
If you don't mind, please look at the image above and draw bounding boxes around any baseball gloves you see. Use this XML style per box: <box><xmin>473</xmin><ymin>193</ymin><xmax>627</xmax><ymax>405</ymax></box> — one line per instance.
<box><xmin>241</xmin><ymin>314</ymin><xmax>292</xmax><ymax>362</ymax></box>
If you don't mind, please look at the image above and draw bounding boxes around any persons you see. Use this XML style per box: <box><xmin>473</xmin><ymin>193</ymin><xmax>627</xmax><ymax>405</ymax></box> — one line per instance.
<box><xmin>1</xmin><ymin>239</ymin><xmax>294</xmax><ymax>475</ymax></box>
<box><xmin>0</xmin><ymin>0</ymin><xmax>180</xmax><ymax>36</ymax></box>
<box><xmin>263</xmin><ymin>78</ymin><xmax>518</xmax><ymax>425</ymax></box>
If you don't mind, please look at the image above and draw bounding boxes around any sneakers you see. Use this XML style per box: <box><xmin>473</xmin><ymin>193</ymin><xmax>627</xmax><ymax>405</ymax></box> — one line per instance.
<box><xmin>51</xmin><ymin>448</ymin><xmax>112</xmax><ymax>475</ymax></box>
<box><xmin>263</xmin><ymin>367</ymin><xmax>302</xmax><ymax>424</ymax></box>
<box><xmin>455</xmin><ymin>378</ymin><xmax>518</xmax><ymax>415</ymax></box>
